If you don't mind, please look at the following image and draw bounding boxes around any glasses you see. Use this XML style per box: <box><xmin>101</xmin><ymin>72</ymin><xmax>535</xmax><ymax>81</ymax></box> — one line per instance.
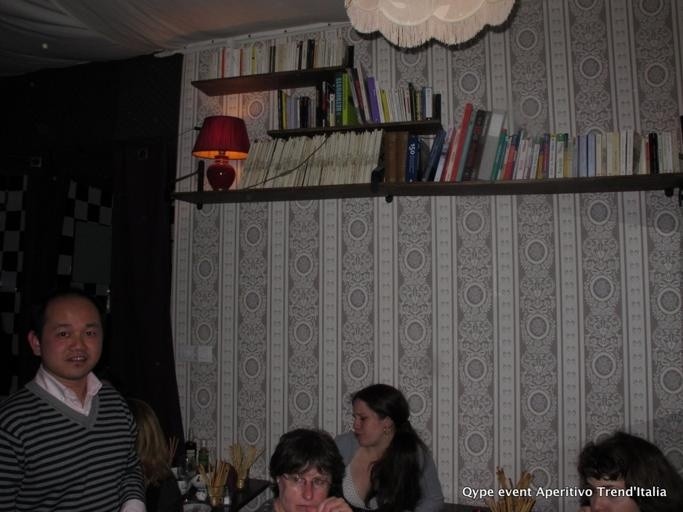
<box><xmin>282</xmin><ymin>473</ymin><xmax>332</xmax><ymax>488</ymax></box>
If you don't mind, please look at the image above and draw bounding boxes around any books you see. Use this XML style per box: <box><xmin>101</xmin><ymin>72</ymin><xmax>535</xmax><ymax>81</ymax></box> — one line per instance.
<box><xmin>205</xmin><ymin>33</ymin><xmax>675</xmax><ymax>189</ymax></box>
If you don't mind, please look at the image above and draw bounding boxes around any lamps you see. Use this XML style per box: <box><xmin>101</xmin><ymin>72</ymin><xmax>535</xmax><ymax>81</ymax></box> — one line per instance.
<box><xmin>192</xmin><ymin>116</ymin><xmax>251</xmax><ymax>191</ymax></box>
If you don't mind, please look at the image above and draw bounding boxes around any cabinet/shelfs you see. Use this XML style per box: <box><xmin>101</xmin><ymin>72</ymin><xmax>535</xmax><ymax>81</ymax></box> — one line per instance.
<box><xmin>173</xmin><ymin>35</ymin><xmax>681</xmax><ymax>211</ymax></box>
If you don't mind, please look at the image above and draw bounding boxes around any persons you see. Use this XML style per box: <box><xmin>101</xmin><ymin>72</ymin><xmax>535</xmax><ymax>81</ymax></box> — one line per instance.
<box><xmin>332</xmin><ymin>382</ymin><xmax>444</xmax><ymax>512</ymax></box>
<box><xmin>121</xmin><ymin>393</ymin><xmax>187</xmax><ymax>512</ymax></box>
<box><xmin>1</xmin><ymin>281</ymin><xmax>151</xmax><ymax>512</ymax></box>
<box><xmin>572</xmin><ymin>429</ymin><xmax>682</xmax><ymax>511</ymax></box>
<box><xmin>253</xmin><ymin>428</ymin><xmax>359</xmax><ymax>512</ymax></box>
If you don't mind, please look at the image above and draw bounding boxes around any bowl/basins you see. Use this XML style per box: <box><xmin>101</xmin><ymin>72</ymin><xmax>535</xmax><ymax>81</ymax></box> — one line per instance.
<box><xmin>170</xmin><ymin>467</ymin><xmax>231</xmax><ymax>512</ymax></box>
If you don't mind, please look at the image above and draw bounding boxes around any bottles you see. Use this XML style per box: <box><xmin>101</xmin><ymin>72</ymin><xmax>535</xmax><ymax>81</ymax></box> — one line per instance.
<box><xmin>198</xmin><ymin>439</ymin><xmax>209</xmax><ymax>471</ymax></box>
<box><xmin>183</xmin><ymin>428</ymin><xmax>196</xmax><ymax>461</ymax></box>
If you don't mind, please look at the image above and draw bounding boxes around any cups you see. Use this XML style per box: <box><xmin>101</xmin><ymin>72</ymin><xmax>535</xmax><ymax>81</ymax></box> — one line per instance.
<box><xmin>235</xmin><ymin>478</ymin><xmax>248</xmax><ymax>490</ymax></box>
